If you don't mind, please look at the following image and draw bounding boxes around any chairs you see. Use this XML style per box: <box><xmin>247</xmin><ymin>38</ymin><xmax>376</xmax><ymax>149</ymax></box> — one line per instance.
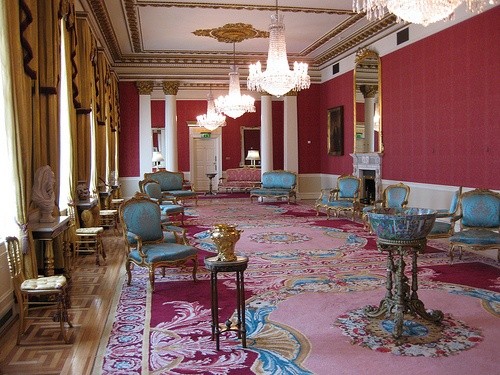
<box><xmin>447</xmin><ymin>188</ymin><xmax>500</xmax><ymax>268</ymax></box>
<box><xmin>426</xmin><ymin>185</ymin><xmax>463</xmax><ymax>239</ymax></box>
<box><xmin>91</xmin><ymin>189</ymin><xmax>120</xmax><ymax>235</ymax></box>
<box><xmin>326</xmin><ymin>174</ymin><xmax>362</xmax><ymax>222</ymax></box>
<box><xmin>111</xmin><ymin>198</ymin><xmax>124</xmax><ymax>224</ymax></box>
<box><xmin>118</xmin><ymin>191</ymin><xmax>198</xmax><ymax>294</ymax></box>
<box><xmin>369</xmin><ymin>182</ymin><xmax>411</xmax><ymax>236</ymax></box>
<box><xmin>5</xmin><ymin>236</ymin><xmax>74</xmax><ymax>346</ymax></box>
<box><xmin>362</xmin><ymin>205</ymin><xmax>377</xmax><ymax>232</ymax></box>
<box><xmin>133</xmin><ymin>190</ymin><xmax>185</xmax><ymax>226</ymax></box>
<box><xmin>138</xmin><ymin>178</ymin><xmax>182</xmax><ymax>204</ymax></box>
<box><xmin>315</xmin><ymin>188</ymin><xmax>335</xmax><ymax>217</ymax></box>
<box><xmin>66</xmin><ymin>202</ymin><xmax>107</xmax><ymax>267</ymax></box>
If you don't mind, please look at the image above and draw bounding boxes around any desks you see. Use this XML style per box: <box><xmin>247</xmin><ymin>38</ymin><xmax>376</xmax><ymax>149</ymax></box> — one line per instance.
<box><xmin>228</xmin><ymin>180</ymin><xmax>261</xmax><ymax>194</ymax></box>
<box><xmin>362</xmin><ymin>238</ymin><xmax>444</xmax><ymax>341</ymax></box>
<box><xmin>203</xmin><ymin>252</ymin><xmax>248</xmax><ymax>353</ymax></box>
<box><xmin>76</xmin><ymin>197</ymin><xmax>98</xmax><ymax>256</ymax></box>
<box><xmin>98</xmin><ymin>192</ymin><xmax>110</xmax><ymax>230</ymax></box>
<box><xmin>31</xmin><ymin>215</ymin><xmax>73</xmax><ymax>309</ymax></box>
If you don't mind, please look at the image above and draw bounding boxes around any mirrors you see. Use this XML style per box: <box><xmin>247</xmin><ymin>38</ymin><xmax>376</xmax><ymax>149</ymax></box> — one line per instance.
<box><xmin>239</xmin><ymin>125</ymin><xmax>260</xmax><ymax>168</ymax></box>
<box><xmin>354</xmin><ymin>47</ymin><xmax>385</xmax><ymax>154</ymax></box>
<box><xmin>152</xmin><ymin>127</ymin><xmax>165</xmax><ymax>168</ymax></box>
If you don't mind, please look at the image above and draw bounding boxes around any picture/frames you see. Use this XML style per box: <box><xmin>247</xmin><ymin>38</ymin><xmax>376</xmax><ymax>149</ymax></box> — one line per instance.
<box><xmin>327</xmin><ymin>105</ymin><xmax>344</xmax><ymax>157</ymax></box>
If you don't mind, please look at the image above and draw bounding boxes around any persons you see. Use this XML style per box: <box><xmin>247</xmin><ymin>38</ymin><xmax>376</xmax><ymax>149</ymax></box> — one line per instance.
<box><xmin>32</xmin><ymin>165</ymin><xmax>56</xmax><ymax>223</ymax></box>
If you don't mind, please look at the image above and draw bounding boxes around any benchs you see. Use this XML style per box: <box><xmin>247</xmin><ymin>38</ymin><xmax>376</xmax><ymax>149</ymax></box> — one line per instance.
<box><xmin>144</xmin><ymin>169</ymin><xmax>199</xmax><ymax>207</ymax></box>
<box><xmin>218</xmin><ymin>168</ymin><xmax>261</xmax><ymax>194</ymax></box>
<box><xmin>249</xmin><ymin>169</ymin><xmax>298</xmax><ymax>205</ymax></box>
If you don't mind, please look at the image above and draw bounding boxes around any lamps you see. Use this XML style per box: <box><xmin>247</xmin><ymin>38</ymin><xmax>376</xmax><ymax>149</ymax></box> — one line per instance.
<box><xmin>249</xmin><ymin>150</ymin><xmax>261</xmax><ymax>168</ymax></box>
<box><xmin>352</xmin><ymin>0</ymin><xmax>500</xmax><ymax>29</ymax></box>
<box><xmin>196</xmin><ymin>83</ymin><xmax>227</xmax><ymax>132</ymax></box>
<box><xmin>246</xmin><ymin>0</ymin><xmax>311</xmax><ymax>99</ymax></box>
<box><xmin>152</xmin><ymin>151</ymin><xmax>165</xmax><ymax>172</ymax></box>
<box><xmin>245</xmin><ymin>150</ymin><xmax>253</xmax><ymax>165</ymax></box>
<box><xmin>214</xmin><ymin>34</ymin><xmax>256</xmax><ymax>120</ymax></box>
